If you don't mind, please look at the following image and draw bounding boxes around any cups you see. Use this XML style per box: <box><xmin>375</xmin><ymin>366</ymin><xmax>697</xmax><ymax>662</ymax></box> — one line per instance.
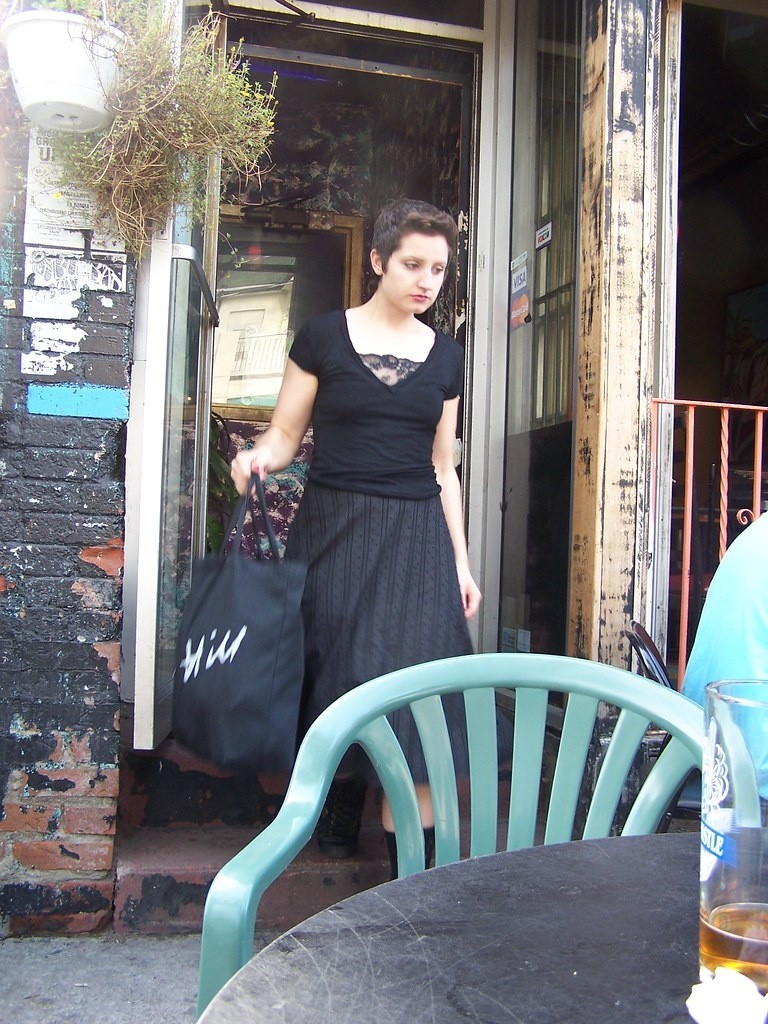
<box><xmin>698</xmin><ymin>680</ymin><xmax>768</xmax><ymax>994</ymax></box>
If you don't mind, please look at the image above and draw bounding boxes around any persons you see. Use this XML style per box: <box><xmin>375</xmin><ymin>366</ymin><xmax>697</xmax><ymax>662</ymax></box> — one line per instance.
<box><xmin>677</xmin><ymin>511</ymin><xmax>768</xmax><ymax>807</ymax></box>
<box><xmin>230</xmin><ymin>200</ymin><xmax>483</xmax><ymax>880</ymax></box>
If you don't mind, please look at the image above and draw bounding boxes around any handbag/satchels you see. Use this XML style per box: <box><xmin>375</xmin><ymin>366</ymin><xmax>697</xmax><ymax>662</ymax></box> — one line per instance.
<box><xmin>171</xmin><ymin>472</ymin><xmax>309</xmax><ymax>779</ymax></box>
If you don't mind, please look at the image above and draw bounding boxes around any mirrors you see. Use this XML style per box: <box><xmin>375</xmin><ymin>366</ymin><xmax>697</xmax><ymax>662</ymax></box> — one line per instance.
<box><xmin>182</xmin><ymin>202</ymin><xmax>364</xmax><ymax>419</ymax></box>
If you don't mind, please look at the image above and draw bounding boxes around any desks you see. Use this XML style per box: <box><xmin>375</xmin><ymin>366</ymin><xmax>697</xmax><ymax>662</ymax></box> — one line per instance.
<box><xmin>200</xmin><ymin>830</ymin><xmax>768</xmax><ymax>1023</ymax></box>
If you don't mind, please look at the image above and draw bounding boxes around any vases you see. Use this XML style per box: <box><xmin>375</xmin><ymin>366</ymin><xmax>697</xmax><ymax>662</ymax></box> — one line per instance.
<box><xmin>1</xmin><ymin>10</ymin><xmax>129</xmax><ymax>134</ymax></box>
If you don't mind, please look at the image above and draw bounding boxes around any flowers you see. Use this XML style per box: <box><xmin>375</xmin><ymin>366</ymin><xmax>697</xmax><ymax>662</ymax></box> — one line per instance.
<box><xmin>0</xmin><ymin>0</ymin><xmax>279</xmax><ymax>265</ymax></box>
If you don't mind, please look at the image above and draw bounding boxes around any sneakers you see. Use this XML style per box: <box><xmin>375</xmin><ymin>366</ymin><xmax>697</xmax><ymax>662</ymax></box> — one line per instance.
<box><xmin>316</xmin><ymin>770</ymin><xmax>368</xmax><ymax>859</ymax></box>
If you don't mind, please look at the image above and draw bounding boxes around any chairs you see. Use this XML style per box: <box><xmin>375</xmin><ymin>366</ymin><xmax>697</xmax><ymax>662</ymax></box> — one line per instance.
<box><xmin>626</xmin><ymin>620</ymin><xmax>703</xmax><ymax>836</ymax></box>
<box><xmin>196</xmin><ymin>651</ymin><xmax>708</xmax><ymax>1018</ymax></box>
